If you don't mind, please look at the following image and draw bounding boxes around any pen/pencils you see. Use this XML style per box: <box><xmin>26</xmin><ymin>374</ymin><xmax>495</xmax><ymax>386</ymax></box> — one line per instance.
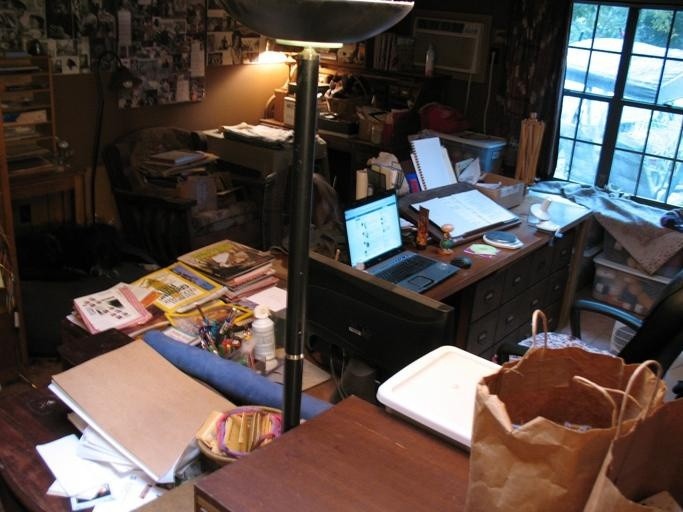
<box><xmin>196</xmin><ymin>304</ymin><xmax>254</xmax><ymax>356</ymax></box>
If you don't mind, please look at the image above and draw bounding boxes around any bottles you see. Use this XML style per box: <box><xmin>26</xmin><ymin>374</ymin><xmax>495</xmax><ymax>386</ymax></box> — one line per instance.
<box><xmin>355</xmin><ymin>169</ymin><xmax>368</xmax><ymax>201</ymax></box>
<box><xmin>57</xmin><ymin>140</ymin><xmax>70</xmax><ymax>164</ymax></box>
<box><xmin>425</xmin><ymin>44</ymin><xmax>436</xmax><ymax>77</ymax></box>
<box><xmin>592</xmin><ymin>268</ymin><xmax>644</xmax><ymax>315</ymax></box>
<box><xmin>252</xmin><ymin>304</ymin><xmax>275</xmax><ymax>361</ymax></box>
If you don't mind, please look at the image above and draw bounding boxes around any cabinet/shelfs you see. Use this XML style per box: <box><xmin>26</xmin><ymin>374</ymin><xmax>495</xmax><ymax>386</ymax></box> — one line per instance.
<box><xmin>273</xmin><ymin>59</ymin><xmax>418</xmax><ymax>205</ymax></box>
<box><xmin>0</xmin><ymin>53</ymin><xmax>60</xmax><ymax>178</ymax></box>
<box><xmin>9</xmin><ymin>167</ymin><xmax>88</xmax><ymax>229</ymax></box>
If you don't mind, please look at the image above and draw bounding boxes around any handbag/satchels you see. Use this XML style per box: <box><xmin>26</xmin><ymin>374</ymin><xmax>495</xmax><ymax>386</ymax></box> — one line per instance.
<box><xmin>582</xmin><ymin>360</ymin><xmax>682</xmax><ymax>511</ymax></box>
<box><xmin>463</xmin><ymin>309</ymin><xmax>667</xmax><ymax>511</ymax></box>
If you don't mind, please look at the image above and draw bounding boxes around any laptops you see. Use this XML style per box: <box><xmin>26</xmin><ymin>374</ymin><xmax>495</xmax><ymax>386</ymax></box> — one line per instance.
<box><xmin>343</xmin><ymin>188</ymin><xmax>462</xmax><ymax>295</ymax></box>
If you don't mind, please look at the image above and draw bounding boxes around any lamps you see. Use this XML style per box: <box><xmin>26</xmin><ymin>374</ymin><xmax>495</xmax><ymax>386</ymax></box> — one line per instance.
<box><xmin>210</xmin><ymin>1</ymin><xmax>411</xmax><ymax>431</ymax></box>
<box><xmin>91</xmin><ymin>48</ymin><xmax>142</xmax><ymax>226</ymax></box>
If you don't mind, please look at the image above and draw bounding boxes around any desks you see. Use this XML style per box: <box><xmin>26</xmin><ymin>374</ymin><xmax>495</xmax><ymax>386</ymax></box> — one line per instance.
<box><xmin>291</xmin><ymin>193</ymin><xmax>591</xmax><ymax>356</ymax></box>
<box><xmin>198</xmin><ymin>123</ymin><xmax>328</xmax><ymax>250</ymax></box>
<box><xmin>194</xmin><ymin>391</ymin><xmax>468</xmax><ymax>511</ymax></box>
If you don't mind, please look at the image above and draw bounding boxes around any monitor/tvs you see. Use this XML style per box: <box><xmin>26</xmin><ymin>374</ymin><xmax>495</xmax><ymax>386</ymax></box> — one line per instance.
<box><xmin>306</xmin><ymin>251</ymin><xmax>455</xmax><ymax>405</ymax></box>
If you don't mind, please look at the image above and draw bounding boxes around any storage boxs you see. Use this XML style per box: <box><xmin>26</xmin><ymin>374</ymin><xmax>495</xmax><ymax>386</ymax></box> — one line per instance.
<box><xmin>421</xmin><ymin>125</ymin><xmax>509</xmax><ymax>176</ymax></box>
<box><xmin>592</xmin><ymin>252</ymin><xmax>675</xmax><ymax>321</ymax></box>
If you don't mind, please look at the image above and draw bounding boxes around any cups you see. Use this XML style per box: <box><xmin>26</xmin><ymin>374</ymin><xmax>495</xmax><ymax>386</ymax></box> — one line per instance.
<box><xmin>21</xmin><ymin>36</ymin><xmax>43</xmax><ymax>57</ymax></box>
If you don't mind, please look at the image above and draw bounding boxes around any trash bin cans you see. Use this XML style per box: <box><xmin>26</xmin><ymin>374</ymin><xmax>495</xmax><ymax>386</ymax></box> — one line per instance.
<box><xmin>20</xmin><ymin>267</ymin><xmax>77</xmax><ymax>360</ymax></box>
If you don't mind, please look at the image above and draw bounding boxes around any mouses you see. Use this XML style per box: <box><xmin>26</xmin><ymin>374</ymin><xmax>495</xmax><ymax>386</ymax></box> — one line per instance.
<box><xmin>450</xmin><ymin>256</ymin><xmax>472</xmax><ymax>268</ymax></box>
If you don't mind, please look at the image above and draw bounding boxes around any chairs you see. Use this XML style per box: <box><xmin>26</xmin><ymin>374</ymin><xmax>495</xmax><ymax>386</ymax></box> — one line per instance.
<box><xmin>497</xmin><ymin>269</ymin><xmax>682</xmax><ymax>375</ymax></box>
<box><xmin>103</xmin><ymin>125</ymin><xmax>276</xmax><ymax>268</ymax></box>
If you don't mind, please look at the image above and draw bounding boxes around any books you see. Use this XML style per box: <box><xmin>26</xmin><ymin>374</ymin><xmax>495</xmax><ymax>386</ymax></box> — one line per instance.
<box><xmin>47</xmin><ymin>338</ymin><xmax>238</xmax><ymax>484</ymax></box>
<box><xmin>410</xmin><ymin>137</ymin><xmax>520</xmax><ymax>237</ymax></box>
<box><xmin>136</xmin><ymin>149</ymin><xmax>220</xmax><ymax>177</ymax></box>
<box><xmin>66</xmin><ymin>239</ymin><xmax>280</xmax><ymax>337</ymax></box>
<box><xmin>0</xmin><ymin>52</ymin><xmax>57</xmax><ymax>179</ymax></box>
<box><xmin>372</xmin><ymin>33</ymin><xmax>405</xmax><ymax>71</ymax></box>
